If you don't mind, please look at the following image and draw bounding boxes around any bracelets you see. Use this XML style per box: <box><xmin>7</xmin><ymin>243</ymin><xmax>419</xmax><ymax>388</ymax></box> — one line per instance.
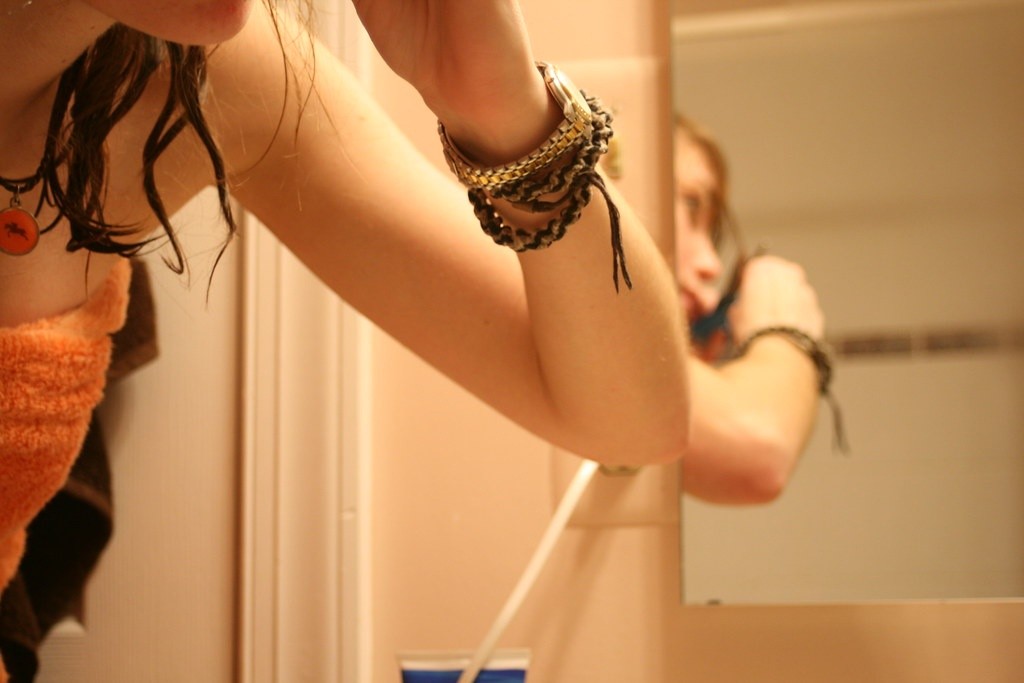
<box><xmin>466</xmin><ymin>89</ymin><xmax>636</xmax><ymax>297</ymax></box>
<box><xmin>733</xmin><ymin>322</ymin><xmax>852</xmax><ymax>453</ymax></box>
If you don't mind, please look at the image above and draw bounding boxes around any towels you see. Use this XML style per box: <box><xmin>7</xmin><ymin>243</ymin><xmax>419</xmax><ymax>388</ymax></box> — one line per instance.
<box><xmin>0</xmin><ymin>250</ymin><xmax>159</xmax><ymax>683</ymax></box>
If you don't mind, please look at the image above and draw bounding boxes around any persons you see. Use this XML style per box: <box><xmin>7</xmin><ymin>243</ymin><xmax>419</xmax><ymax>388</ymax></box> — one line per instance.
<box><xmin>1</xmin><ymin>0</ymin><xmax>695</xmax><ymax>683</ymax></box>
<box><xmin>664</xmin><ymin>104</ymin><xmax>835</xmax><ymax>509</ymax></box>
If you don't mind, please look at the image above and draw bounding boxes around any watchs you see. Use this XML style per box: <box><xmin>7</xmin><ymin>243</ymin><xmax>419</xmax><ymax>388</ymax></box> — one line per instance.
<box><xmin>435</xmin><ymin>61</ymin><xmax>593</xmax><ymax>191</ymax></box>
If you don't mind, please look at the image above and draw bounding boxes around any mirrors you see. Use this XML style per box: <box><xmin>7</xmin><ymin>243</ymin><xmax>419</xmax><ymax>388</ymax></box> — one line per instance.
<box><xmin>235</xmin><ymin>0</ymin><xmax>1024</xmax><ymax>683</ymax></box>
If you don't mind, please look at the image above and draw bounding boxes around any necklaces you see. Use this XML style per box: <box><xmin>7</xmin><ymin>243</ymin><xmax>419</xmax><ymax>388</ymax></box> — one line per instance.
<box><xmin>0</xmin><ymin>126</ymin><xmax>76</xmax><ymax>262</ymax></box>
